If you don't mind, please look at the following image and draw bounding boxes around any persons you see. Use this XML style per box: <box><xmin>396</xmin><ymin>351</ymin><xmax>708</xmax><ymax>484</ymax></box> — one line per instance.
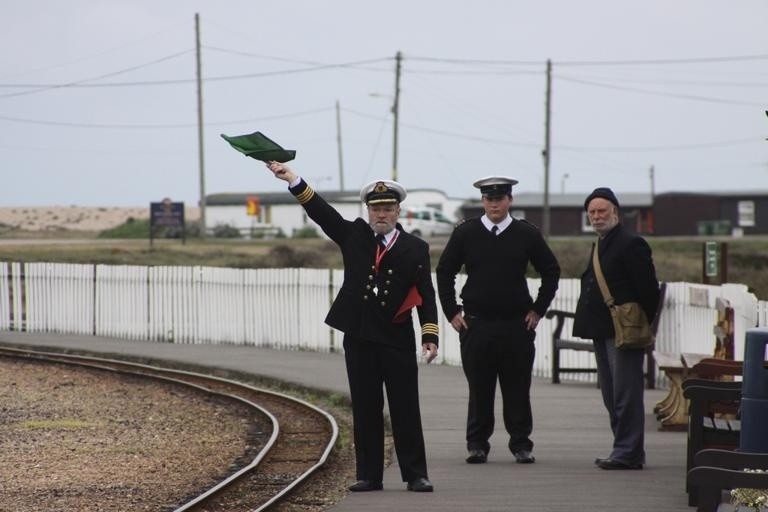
<box><xmin>572</xmin><ymin>188</ymin><xmax>659</xmax><ymax>470</ymax></box>
<box><xmin>265</xmin><ymin>159</ymin><xmax>439</xmax><ymax>492</ymax></box>
<box><xmin>436</xmin><ymin>177</ymin><xmax>560</xmax><ymax>464</ymax></box>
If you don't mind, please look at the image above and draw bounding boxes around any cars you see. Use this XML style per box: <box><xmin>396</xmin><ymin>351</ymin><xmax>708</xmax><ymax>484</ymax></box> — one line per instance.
<box><xmin>391</xmin><ymin>204</ymin><xmax>458</xmax><ymax>236</ymax></box>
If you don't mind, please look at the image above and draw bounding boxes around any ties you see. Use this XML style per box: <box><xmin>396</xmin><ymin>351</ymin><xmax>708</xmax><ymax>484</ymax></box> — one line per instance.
<box><xmin>376</xmin><ymin>234</ymin><xmax>387</xmax><ymax>256</ymax></box>
<box><xmin>492</xmin><ymin>226</ymin><xmax>498</xmax><ymax>235</ymax></box>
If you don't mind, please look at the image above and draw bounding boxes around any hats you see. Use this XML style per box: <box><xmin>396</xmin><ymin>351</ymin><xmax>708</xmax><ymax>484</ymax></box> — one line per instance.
<box><xmin>361</xmin><ymin>180</ymin><xmax>407</xmax><ymax>207</ymax></box>
<box><xmin>473</xmin><ymin>176</ymin><xmax>518</xmax><ymax>200</ymax></box>
<box><xmin>584</xmin><ymin>189</ymin><xmax>620</xmax><ymax>213</ymax></box>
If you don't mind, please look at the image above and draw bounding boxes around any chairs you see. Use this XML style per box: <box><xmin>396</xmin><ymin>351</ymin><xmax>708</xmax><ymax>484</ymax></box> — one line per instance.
<box><xmin>691</xmin><ymin>357</ymin><xmax>768</xmax><ymax>419</ymax></box>
<box><xmin>546</xmin><ymin>279</ymin><xmax>666</xmax><ymax>389</ymax></box>
<box><xmin>681</xmin><ymin>378</ymin><xmax>741</xmax><ymax>507</ymax></box>
<box><xmin>685</xmin><ymin>448</ymin><xmax>768</xmax><ymax>512</ymax></box>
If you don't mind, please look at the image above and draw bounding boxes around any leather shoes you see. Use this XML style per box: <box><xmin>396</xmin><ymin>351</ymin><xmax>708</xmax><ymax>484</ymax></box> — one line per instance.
<box><xmin>406</xmin><ymin>476</ymin><xmax>434</xmax><ymax>492</ymax></box>
<box><xmin>598</xmin><ymin>459</ymin><xmax>644</xmax><ymax>471</ymax></box>
<box><xmin>594</xmin><ymin>456</ymin><xmax>610</xmax><ymax>468</ymax></box>
<box><xmin>465</xmin><ymin>450</ymin><xmax>488</xmax><ymax>464</ymax></box>
<box><xmin>515</xmin><ymin>450</ymin><xmax>537</xmax><ymax>464</ymax></box>
<box><xmin>348</xmin><ymin>479</ymin><xmax>384</xmax><ymax>492</ymax></box>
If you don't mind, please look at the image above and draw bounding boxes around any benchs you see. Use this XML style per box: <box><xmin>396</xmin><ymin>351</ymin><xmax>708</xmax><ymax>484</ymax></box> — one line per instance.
<box><xmin>654</xmin><ymin>297</ymin><xmax>734</xmax><ymax>436</ymax></box>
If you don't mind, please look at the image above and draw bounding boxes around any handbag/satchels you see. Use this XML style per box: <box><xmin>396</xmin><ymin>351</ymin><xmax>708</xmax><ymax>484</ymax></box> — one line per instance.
<box><xmin>592</xmin><ymin>236</ymin><xmax>655</xmax><ymax>351</ymax></box>
